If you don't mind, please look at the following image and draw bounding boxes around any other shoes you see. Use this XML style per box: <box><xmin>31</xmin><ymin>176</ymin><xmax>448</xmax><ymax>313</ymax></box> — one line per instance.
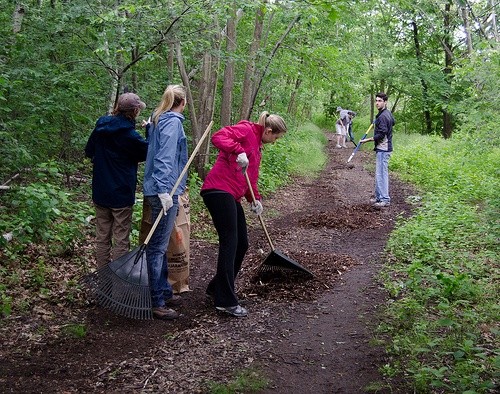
<box><xmin>370</xmin><ymin>198</ymin><xmax>378</xmax><ymax>204</ymax></box>
<box><xmin>336</xmin><ymin>144</ymin><xmax>341</xmax><ymax>148</ymax></box>
<box><xmin>343</xmin><ymin>145</ymin><xmax>348</xmax><ymax>149</ymax></box>
<box><xmin>373</xmin><ymin>201</ymin><xmax>390</xmax><ymax>207</ymax></box>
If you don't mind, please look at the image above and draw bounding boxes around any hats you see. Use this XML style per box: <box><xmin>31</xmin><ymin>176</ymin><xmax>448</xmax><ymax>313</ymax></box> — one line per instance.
<box><xmin>118</xmin><ymin>92</ymin><xmax>146</xmax><ymax>110</ymax></box>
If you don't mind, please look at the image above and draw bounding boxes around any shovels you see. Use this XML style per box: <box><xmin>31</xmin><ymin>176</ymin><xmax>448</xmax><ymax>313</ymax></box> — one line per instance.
<box><xmin>333</xmin><ymin>112</ymin><xmax>357</xmax><ymax>147</ymax></box>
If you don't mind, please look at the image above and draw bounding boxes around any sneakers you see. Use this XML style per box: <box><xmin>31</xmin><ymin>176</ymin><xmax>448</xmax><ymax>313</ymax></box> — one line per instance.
<box><xmin>216</xmin><ymin>305</ymin><xmax>247</xmax><ymax>316</ymax></box>
<box><xmin>165</xmin><ymin>295</ymin><xmax>180</xmax><ymax>304</ymax></box>
<box><xmin>141</xmin><ymin>306</ymin><xmax>178</xmax><ymax>319</ymax></box>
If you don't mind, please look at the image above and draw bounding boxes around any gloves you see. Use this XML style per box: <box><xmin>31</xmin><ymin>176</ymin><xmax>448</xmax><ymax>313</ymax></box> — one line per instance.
<box><xmin>158</xmin><ymin>192</ymin><xmax>173</xmax><ymax>215</ymax></box>
<box><xmin>251</xmin><ymin>199</ymin><xmax>263</xmax><ymax>216</ymax></box>
<box><xmin>236</xmin><ymin>152</ymin><xmax>249</xmax><ymax>174</ymax></box>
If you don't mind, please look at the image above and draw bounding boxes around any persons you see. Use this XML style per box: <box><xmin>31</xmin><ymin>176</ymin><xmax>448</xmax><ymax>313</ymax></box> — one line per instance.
<box><xmin>358</xmin><ymin>93</ymin><xmax>395</xmax><ymax>207</ymax></box>
<box><xmin>336</xmin><ymin>106</ymin><xmax>355</xmax><ymax>142</ymax></box>
<box><xmin>199</xmin><ymin>110</ymin><xmax>287</xmax><ymax>318</ymax></box>
<box><xmin>82</xmin><ymin>91</ymin><xmax>151</xmax><ymax>309</ymax></box>
<box><xmin>334</xmin><ymin>112</ymin><xmax>356</xmax><ymax>149</ymax></box>
<box><xmin>140</xmin><ymin>83</ymin><xmax>194</xmax><ymax>317</ymax></box>
